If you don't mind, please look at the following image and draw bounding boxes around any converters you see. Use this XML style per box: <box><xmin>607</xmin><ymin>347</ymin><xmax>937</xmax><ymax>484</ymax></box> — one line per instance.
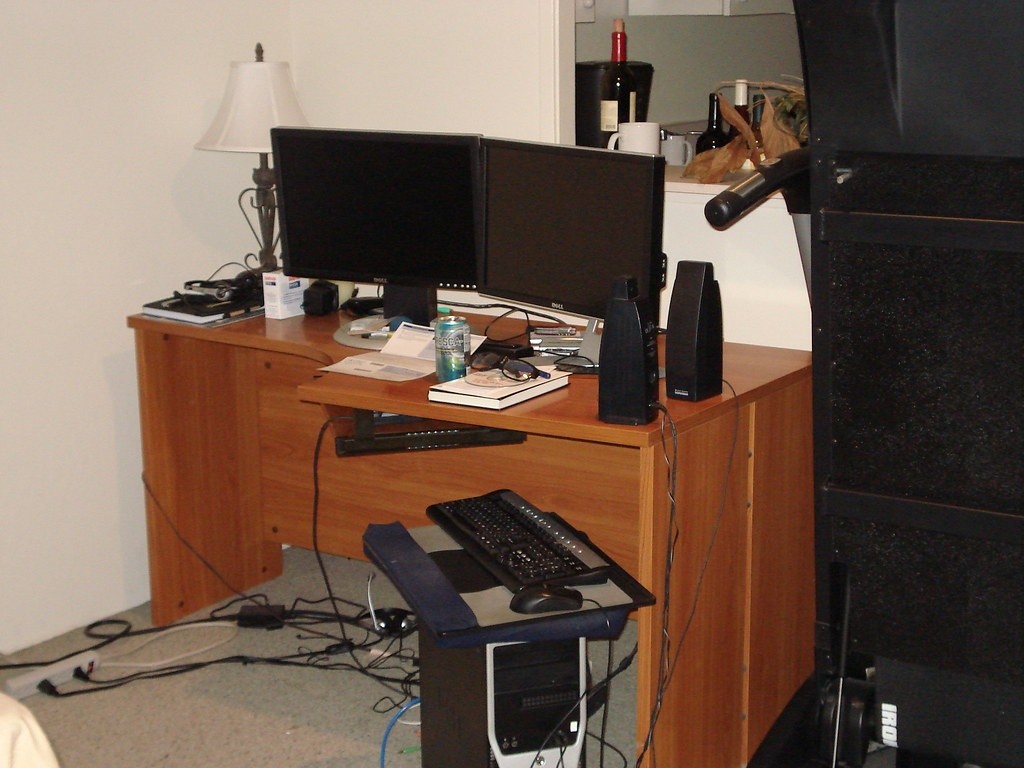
<box><xmin>237</xmin><ymin>604</ymin><xmax>285</xmax><ymax>628</ymax></box>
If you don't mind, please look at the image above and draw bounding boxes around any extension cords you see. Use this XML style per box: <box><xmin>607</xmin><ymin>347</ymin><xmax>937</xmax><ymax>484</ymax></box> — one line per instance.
<box><xmin>6</xmin><ymin>650</ymin><xmax>98</xmax><ymax>697</ymax></box>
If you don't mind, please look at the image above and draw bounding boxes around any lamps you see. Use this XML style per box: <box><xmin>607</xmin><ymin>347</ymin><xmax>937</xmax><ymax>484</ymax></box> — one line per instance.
<box><xmin>193</xmin><ymin>42</ymin><xmax>312</xmax><ymax>279</ymax></box>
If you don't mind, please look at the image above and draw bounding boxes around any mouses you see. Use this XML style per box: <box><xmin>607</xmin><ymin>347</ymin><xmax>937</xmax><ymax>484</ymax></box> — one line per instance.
<box><xmin>511</xmin><ymin>583</ymin><xmax>585</xmax><ymax>614</ymax></box>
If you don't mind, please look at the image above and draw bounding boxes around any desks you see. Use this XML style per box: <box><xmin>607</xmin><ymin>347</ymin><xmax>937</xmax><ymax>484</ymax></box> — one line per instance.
<box><xmin>125</xmin><ymin>294</ymin><xmax>813</xmax><ymax>768</ymax></box>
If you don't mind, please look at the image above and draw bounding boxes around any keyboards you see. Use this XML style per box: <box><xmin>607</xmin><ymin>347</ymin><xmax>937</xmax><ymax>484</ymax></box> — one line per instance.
<box><xmin>427</xmin><ymin>489</ymin><xmax>611</xmax><ymax>591</ymax></box>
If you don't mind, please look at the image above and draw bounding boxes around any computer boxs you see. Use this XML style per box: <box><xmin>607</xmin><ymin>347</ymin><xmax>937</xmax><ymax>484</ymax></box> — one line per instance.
<box><xmin>415</xmin><ymin>615</ymin><xmax>587</xmax><ymax>768</ymax></box>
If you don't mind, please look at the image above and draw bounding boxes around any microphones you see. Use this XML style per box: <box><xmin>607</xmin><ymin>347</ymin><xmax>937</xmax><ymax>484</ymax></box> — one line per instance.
<box><xmin>366</xmin><ymin>573</ymin><xmax>384</xmax><ymax>634</ymax></box>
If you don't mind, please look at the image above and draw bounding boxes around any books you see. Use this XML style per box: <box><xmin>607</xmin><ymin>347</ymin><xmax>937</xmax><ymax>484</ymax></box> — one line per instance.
<box><xmin>141</xmin><ymin>294</ymin><xmax>224</xmax><ymax>325</ymax></box>
<box><xmin>426</xmin><ymin>365</ymin><xmax>573</xmax><ymax>410</ymax></box>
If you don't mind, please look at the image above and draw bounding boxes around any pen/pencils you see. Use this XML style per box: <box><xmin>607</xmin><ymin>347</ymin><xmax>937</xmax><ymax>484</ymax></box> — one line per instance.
<box><xmin>504</xmin><ymin>362</ymin><xmax>550</xmax><ymax>379</ymax></box>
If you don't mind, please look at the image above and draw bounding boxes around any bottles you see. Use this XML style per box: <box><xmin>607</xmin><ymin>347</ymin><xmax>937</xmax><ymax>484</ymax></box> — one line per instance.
<box><xmin>726</xmin><ymin>80</ymin><xmax>748</xmax><ymax>146</ymax></box>
<box><xmin>748</xmin><ymin>94</ymin><xmax>765</xmax><ymax>150</ymax></box>
<box><xmin>695</xmin><ymin>92</ymin><xmax>727</xmax><ymax>154</ymax></box>
<box><xmin>600</xmin><ymin>19</ymin><xmax>637</xmax><ymax>150</ymax></box>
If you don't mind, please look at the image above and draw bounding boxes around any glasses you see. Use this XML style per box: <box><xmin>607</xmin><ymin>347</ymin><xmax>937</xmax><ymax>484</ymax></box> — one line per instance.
<box><xmin>471</xmin><ymin>350</ymin><xmax>553</xmax><ymax>382</ymax></box>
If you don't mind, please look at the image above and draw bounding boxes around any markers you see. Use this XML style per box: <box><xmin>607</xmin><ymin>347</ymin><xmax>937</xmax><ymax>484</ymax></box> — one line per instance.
<box><xmin>361</xmin><ymin>331</ymin><xmax>394</xmax><ymax>338</ymax></box>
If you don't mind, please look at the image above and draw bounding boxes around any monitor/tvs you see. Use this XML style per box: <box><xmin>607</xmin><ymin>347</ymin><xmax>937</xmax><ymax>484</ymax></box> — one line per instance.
<box><xmin>476</xmin><ymin>134</ymin><xmax>664</xmax><ymax>370</ymax></box>
<box><xmin>269</xmin><ymin>125</ymin><xmax>483</xmax><ymax>334</ymax></box>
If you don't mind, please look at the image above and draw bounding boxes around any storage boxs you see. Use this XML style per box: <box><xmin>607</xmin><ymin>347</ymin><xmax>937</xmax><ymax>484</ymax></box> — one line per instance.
<box><xmin>261</xmin><ymin>269</ymin><xmax>308</xmax><ymax>319</ymax></box>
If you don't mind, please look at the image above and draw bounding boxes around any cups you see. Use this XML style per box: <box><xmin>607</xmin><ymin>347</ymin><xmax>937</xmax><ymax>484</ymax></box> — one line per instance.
<box><xmin>608</xmin><ymin>120</ymin><xmax>660</xmax><ymax>155</ymax></box>
<box><xmin>661</xmin><ymin>134</ymin><xmax>692</xmax><ymax>166</ymax></box>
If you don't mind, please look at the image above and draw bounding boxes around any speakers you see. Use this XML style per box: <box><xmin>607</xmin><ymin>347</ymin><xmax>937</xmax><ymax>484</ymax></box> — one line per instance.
<box><xmin>597</xmin><ymin>292</ymin><xmax>661</xmax><ymax>425</ymax></box>
<box><xmin>664</xmin><ymin>260</ymin><xmax>723</xmax><ymax>402</ymax></box>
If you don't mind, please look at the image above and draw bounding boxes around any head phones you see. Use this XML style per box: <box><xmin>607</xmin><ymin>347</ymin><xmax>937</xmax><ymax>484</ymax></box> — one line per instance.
<box><xmin>360</xmin><ymin>607</ymin><xmax>421</xmax><ymax>638</ymax></box>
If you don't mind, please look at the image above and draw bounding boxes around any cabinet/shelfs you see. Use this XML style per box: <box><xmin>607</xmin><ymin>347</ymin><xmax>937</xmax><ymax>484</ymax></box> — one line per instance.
<box><xmin>628</xmin><ymin>0</ymin><xmax>794</xmax><ymax>16</ymax></box>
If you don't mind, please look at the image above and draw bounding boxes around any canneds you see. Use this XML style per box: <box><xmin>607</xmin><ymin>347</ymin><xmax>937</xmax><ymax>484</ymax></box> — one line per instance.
<box><xmin>434</xmin><ymin>315</ymin><xmax>471</xmax><ymax>383</ymax></box>
